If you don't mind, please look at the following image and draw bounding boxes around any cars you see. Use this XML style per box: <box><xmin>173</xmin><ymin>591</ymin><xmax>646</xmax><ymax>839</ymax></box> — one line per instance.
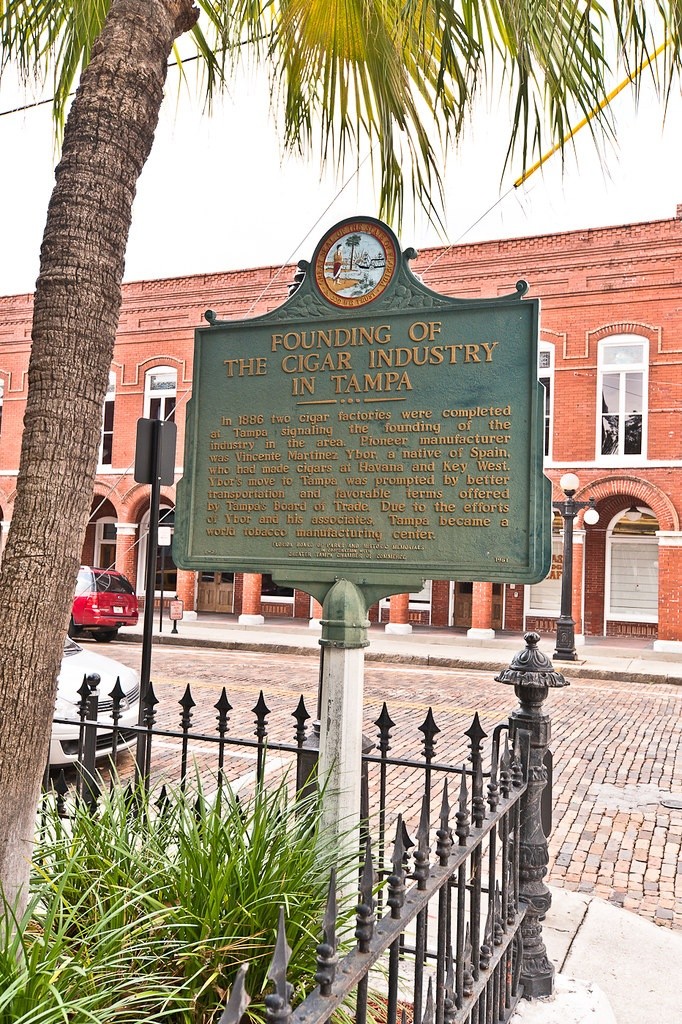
<box><xmin>47</xmin><ymin>636</ymin><xmax>139</xmax><ymax>771</ymax></box>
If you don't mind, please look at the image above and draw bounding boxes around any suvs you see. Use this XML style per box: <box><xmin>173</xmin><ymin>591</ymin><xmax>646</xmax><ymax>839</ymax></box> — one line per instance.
<box><xmin>68</xmin><ymin>565</ymin><xmax>139</xmax><ymax>643</ymax></box>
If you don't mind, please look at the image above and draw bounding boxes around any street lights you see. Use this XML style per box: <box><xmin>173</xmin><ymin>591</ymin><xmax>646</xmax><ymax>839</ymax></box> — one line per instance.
<box><xmin>550</xmin><ymin>473</ymin><xmax>600</xmax><ymax>665</ymax></box>
